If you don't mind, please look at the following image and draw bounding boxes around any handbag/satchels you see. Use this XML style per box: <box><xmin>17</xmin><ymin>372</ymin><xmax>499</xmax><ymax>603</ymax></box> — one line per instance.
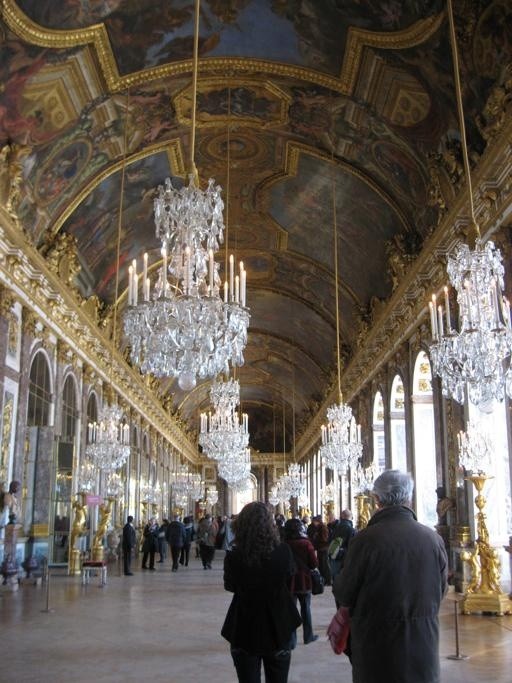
<box><xmin>326</xmin><ymin>606</ymin><xmax>351</xmax><ymax>655</ymax></box>
<box><xmin>311</xmin><ymin>570</ymin><xmax>325</xmax><ymax>595</ymax></box>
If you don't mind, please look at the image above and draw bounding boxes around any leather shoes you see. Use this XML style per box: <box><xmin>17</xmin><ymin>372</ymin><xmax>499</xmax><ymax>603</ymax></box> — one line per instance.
<box><xmin>304</xmin><ymin>635</ymin><xmax>318</xmax><ymax>644</ymax></box>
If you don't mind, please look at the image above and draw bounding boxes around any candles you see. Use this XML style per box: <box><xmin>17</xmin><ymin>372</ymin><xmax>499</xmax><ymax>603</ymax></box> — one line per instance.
<box><xmin>197</xmin><ymin>360</ymin><xmax>251</xmax><ymax>462</ymax></box>
<box><xmin>216</xmin><ymin>449</ymin><xmax>252</xmax><ymax>489</ymax></box>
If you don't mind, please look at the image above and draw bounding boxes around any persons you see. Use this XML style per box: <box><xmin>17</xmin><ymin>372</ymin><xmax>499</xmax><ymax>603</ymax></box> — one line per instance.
<box><xmin>121</xmin><ymin>515</ymin><xmax>141</xmax><ymax>577</ymax></box>
<box><xmin>218</xmin><ymin>504</ymin><xmax>304</xmax><ymax>683</ymax></box>
<box><xmin>277</xmin><ymin>515</ymin><xmax>326</xmax><ymax>650</ymax></box>
<box><xmin>198</xmin><ymin>508</ymin><xmax>218</xmax><ymax>571</ymax></box>
<box><xmin>325</xmin><ymin>510</ymin><xmax>340</xmax><ymax>540</ymax></box>
<box><xmin>324</xmin><ymin>503</ymin><xmax>359</xmax><ymax>575</ymax></box>
<box><xmin>189</xmin><ymin>514</ymin><xmax>195</xmax><ymax>542</ymax></box>
<box><xmin>219</xmin><ymin>514</ymin><xmax>237</xmax><ymax>549</ymax></box>
<box><xmin>2</xmin><ymin>476</ymin><xmax>25</xmax><ymax>568</ymax></box>
<box><xmin>302</xmin><ymin>511</ymin><xmax>330</xmax><ymax>577</ymax></box>
<box><xmin>158</xmin><ymin>516</ymin><xmax>169</xmax><ymax>564</ymax></box>
<box><xmin>167</xmin><ymin>512</ymin><xmax>187</xmax><ymax>571</ymax></box>
<box><xmin>433</xmin><ymin>484</ymin><xmax>456</xmax><ymax>528</ymax></box>
<box><xmin>326</xmin><ymin>470</ymin><xmax>452</xmax><ymax>683</ymax></box>
<box><xmin>274</xmin><ymin>510</ymin><xmax>287</xmax><ymax>541</ymax></box>
<box><xmin>180</xmin><ymin>516</ymin><xmax>196</xmax><ymax>567</ymax></box>
<box><xmin>141</xmin><ymin>516</ymin><xmax>161</xmax><ymax>571</ymax></box>
<box><xmin>298</xmin><ymin>513</ymin><xmax>310</xmax><ymax>536</ymax></box>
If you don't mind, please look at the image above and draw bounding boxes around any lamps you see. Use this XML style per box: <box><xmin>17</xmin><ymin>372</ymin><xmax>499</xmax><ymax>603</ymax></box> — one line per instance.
<box><xmin>317</xmin><ymin>403</ymin><xmax>364</xmax><ymax>518</ymax></box>
<box><xmin>83</xmin><ymin>401</ymin><xmax>132</xmax><ymax>477</ymax></box>
<box><xmin>280</xmin><ymin>460</ymin><xmax>304</xmax><ymax>519</ymax></box>
<box><xmin>121</xmin><ymin>172</ymin><xmax>253</xmax><ymax>393</ymax></box>
<box><xmin>424</xmin><ymin>241</ymin><xmax>512</xmax><ymax>414</ymax></box>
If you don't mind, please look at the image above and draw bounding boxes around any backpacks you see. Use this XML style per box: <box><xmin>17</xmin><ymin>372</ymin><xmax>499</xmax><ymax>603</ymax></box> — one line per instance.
<box><xmin>326</xmin><ymin>525</ymin><xmax>352</xmax><ymax>563</ymax></box>
<box><xmin>198</xmin><ymin>522</ymin><xmax>214</xmax><ymax>547</ymax></box>
